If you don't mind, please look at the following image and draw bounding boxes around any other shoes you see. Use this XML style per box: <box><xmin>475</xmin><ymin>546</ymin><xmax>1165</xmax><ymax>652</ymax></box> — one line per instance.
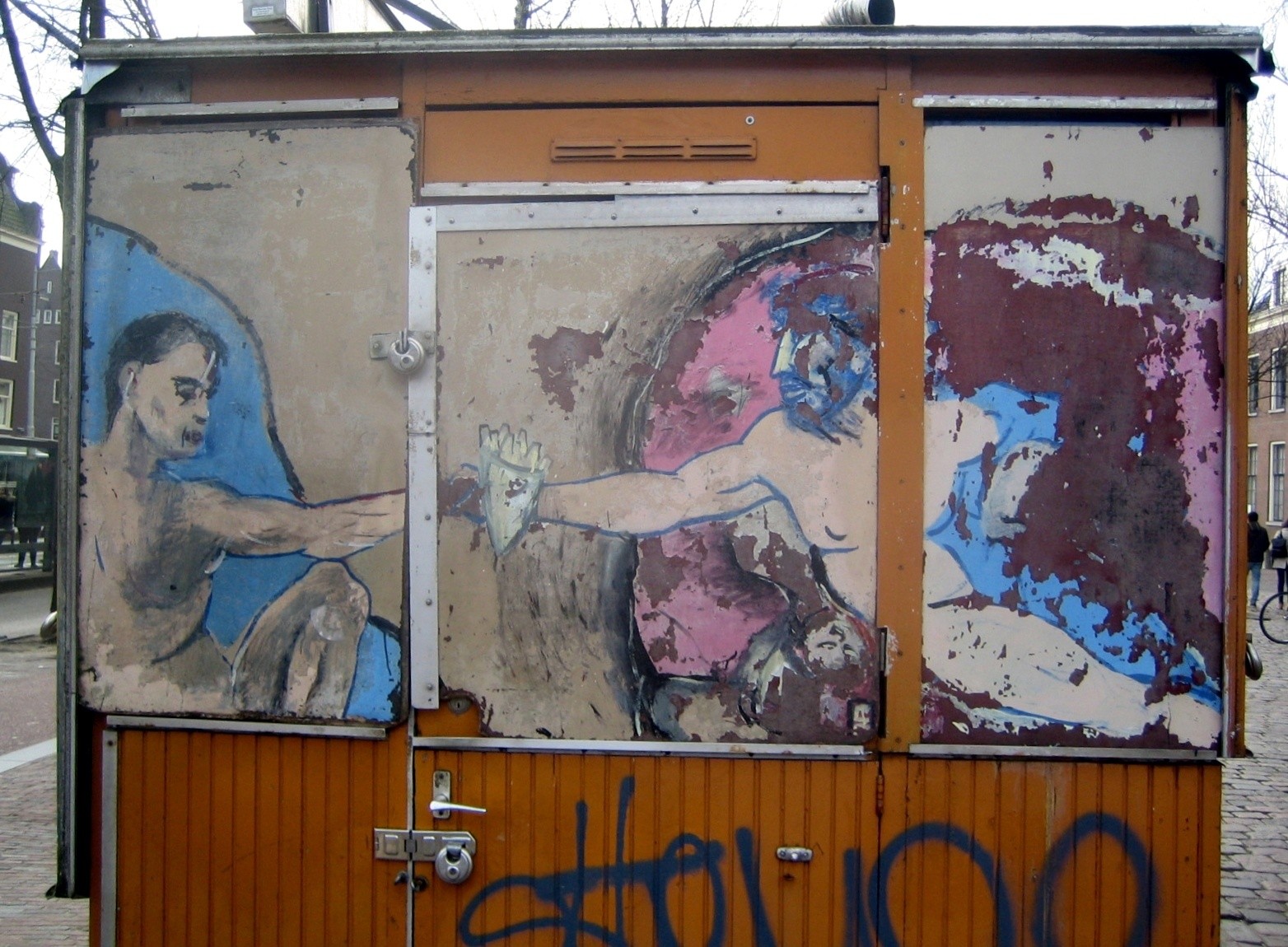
<box><xmin>14</xmin><ymin>563</ymin><xmax>20</xmax><ymax>567</ymax></box>
<box><xmin>1277</xmin><ymin>604</ymin><xmax>1283</xmax><ymax>610</ymax></box>
<box><xmin>30</xmin><ymin>565</ymin><xmax>40</xmax><ymax>570</ymax></box>
<box><xmin>1250</xmin><ymin>602</ymin><xmax>1255</xmax><ymax>608</ymax></box>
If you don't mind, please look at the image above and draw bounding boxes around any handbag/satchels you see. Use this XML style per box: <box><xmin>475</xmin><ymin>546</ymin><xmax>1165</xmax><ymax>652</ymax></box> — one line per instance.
<box><xmin>1264</xmin><ymin>550</ymin><xmax>1273</xmax><ymax>569</ymax></box>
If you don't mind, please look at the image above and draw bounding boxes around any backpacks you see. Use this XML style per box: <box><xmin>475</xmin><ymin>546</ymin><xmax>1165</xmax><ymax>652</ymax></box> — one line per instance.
<box><xmin>1272</xmin><ymin>532</ymin><xmax>1286</xmax><ymax>558</ymax></box>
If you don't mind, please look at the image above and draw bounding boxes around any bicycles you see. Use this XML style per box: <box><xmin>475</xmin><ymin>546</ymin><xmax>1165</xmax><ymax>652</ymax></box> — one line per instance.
<box><xmin>1258</xmin><ymin>591</ymin><xmax>1288</xmax><ymax>645</ymax></box>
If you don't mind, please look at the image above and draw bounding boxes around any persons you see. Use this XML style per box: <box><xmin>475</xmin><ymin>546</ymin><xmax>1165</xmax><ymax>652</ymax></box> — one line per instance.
<box><xmin>14</xmin><ymin>459</ymin><xmax>44</xmax><ymax>567</ymax></box>
<box><xmin>1247</xmin><ymin>512</ymin><xmax>1270</xmax><ymax>608</ymax></box>
<box><xmin>1269</xmin><ymin>519</ymin><xmax>1288</xmax><ymax>611</ymax></box>
